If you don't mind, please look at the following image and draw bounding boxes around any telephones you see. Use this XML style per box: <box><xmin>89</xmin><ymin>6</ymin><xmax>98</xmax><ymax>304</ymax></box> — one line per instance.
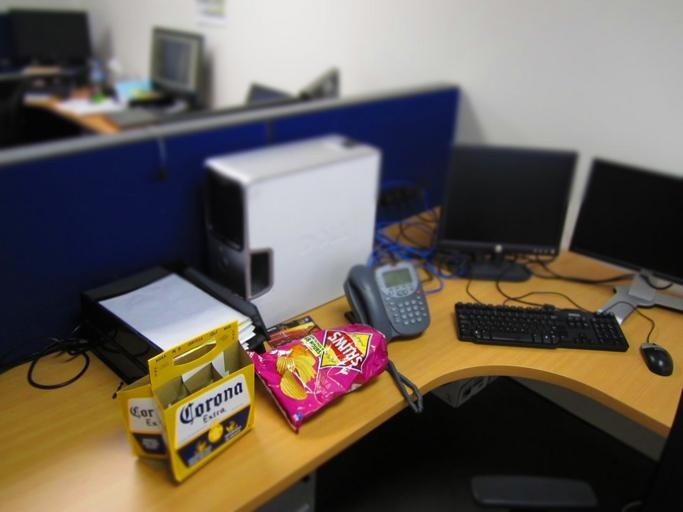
<box><xmin>342</xmin><ymin>261</ymin><xmax>431</xmax><ymax>413</ymax></box>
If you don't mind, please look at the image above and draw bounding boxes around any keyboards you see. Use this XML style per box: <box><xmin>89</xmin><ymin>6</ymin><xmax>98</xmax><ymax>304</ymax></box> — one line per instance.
<box><xmin>455</xmin><ymin>302</ymin><xmax>630</xmax><ymax>354</ymax></box>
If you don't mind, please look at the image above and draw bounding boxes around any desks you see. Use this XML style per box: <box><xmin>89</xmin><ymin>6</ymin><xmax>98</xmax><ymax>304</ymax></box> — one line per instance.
<box><xmin>1</xmin><ymin>206</ymin><xmax>683</xmax><ymax>511</ymax></box>
<box><xmin>23</xmin><ymin>84</ymin><xmax>121</xmax><ymax>136</ymax></box>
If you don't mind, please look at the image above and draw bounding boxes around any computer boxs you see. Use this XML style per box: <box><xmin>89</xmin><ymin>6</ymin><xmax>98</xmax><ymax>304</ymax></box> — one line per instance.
<box><xmin>200</xmin><ymin>131</ymin><xmax>382</xmax><ymax>328</ymax></box>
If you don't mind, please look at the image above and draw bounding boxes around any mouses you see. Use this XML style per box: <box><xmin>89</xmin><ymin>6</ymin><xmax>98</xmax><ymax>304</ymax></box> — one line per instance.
<box><xmin>639</xmin><ymin>342</ymin><xmax>674</xmax><ymax>377</ymax></box>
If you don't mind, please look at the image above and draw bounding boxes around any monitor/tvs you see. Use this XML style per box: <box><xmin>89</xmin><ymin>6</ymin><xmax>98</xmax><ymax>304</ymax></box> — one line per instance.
<box><xmin>436</xmin><ymin>142</ymin><xmax>580</xmax><ymax>282</ymax></box>
<box><xmin>128</xmin><ymin>26</ymin><xmax>201</xmax><ymax>110</ymax></box>
<box><xmin>567</xmin><ymin>156</ymin><xmax>683</xmax><ymax>326</ymax></box>
